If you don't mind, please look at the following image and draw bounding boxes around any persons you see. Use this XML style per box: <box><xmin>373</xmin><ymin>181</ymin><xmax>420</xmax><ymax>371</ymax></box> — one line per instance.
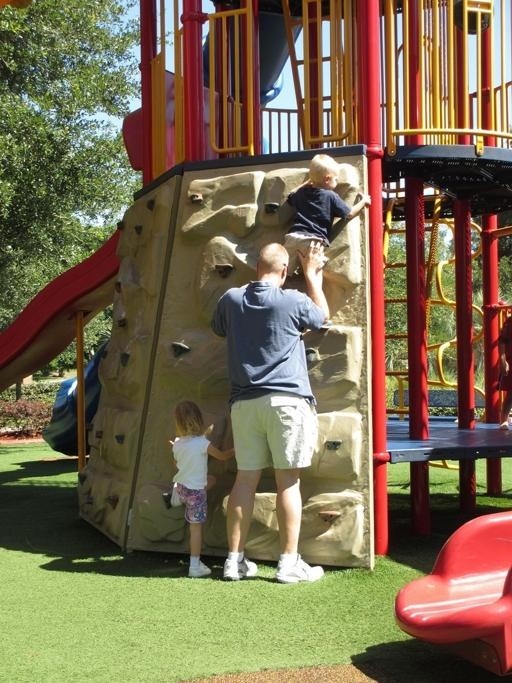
<box><xmin>283</xmin><ymin>154</ymin><xmax>373</xmax><ymax>323</ymax></box>
<box><xmin>208</xmin><ymin>241</ymin><xmax>332</xmax><ymax>583</ymax></box>
<box><xmin>168</xmin><ymin>400</ymin><xmax>234</xmax><ymax>577</ymax></box>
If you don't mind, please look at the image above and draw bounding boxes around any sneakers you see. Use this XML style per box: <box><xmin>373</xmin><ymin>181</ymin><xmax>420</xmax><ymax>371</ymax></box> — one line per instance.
<box><xmin>275</xmin><ymin>554</ymin><xmax>324</xmax><ymax>584</ymax></box>
<box><xmin>320</xmin><ymin>319</ymin><xmax>332</xmax><ymax>328</ymax></box>
<box><xmin>223</xmin><ymin>557</ymin><xmax>258</xmax><ymax>581</ymax></box>
<box><xmin>170</xmin><ymin>482</ymin><xmax>183</xmax><ymax>507</ymax></box>
<box><xmin>188</xmin><ymin>561</ymin><xmax>212</xmax><ymax>577</ymax></box>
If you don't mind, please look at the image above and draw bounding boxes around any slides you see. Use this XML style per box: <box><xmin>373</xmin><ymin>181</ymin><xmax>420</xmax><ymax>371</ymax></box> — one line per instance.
<box><xmin>0</xmin><ymin>226</ymin><xmax>121</xmax><ymax>394</ymax></box>
